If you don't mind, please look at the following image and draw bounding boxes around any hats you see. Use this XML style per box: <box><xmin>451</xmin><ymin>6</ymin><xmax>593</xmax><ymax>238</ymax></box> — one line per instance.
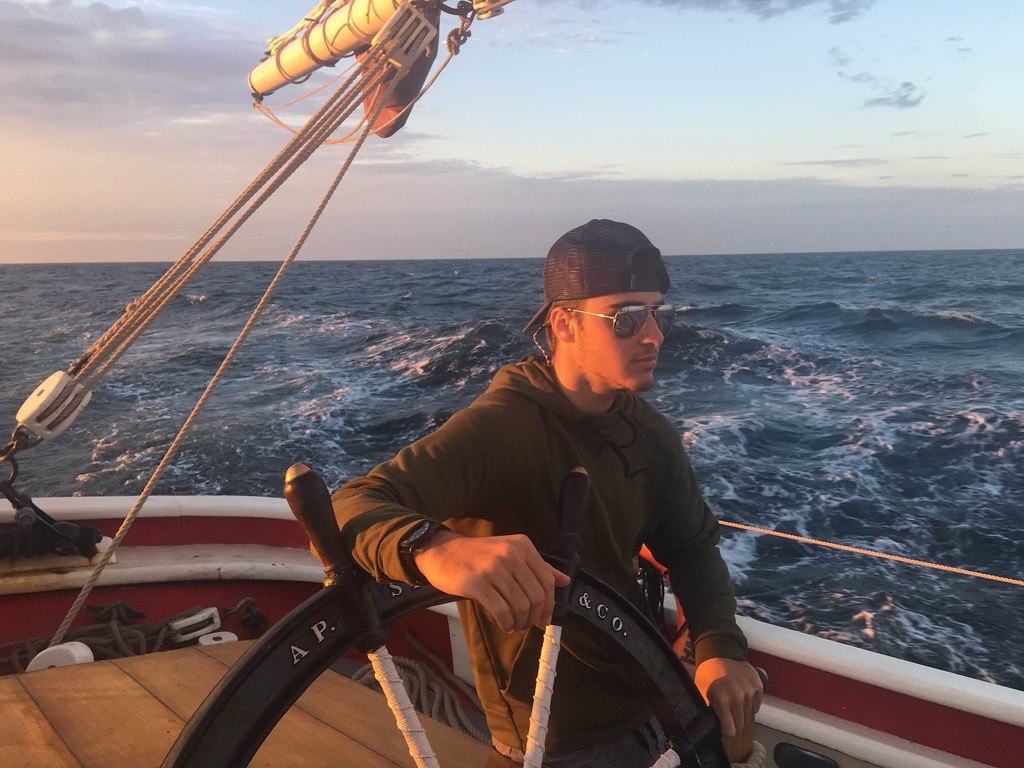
<box><xmin>522</xmin><ymin>219</ymin><xmax>670</xmax><ymax>336</ymax></box>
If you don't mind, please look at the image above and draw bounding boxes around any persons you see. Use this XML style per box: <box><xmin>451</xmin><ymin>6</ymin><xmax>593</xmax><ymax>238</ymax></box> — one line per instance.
<box><xmin>312</xmin><ymin>218</ymin><xmax>764</xmax><ymax>768</ymax></box>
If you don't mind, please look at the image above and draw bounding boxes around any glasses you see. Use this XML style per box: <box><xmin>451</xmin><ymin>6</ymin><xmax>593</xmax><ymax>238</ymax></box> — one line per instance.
<box><xmin>563</xmin><ymin>304</ymin><xmax>678</xmax><ymax>340</ymax></box>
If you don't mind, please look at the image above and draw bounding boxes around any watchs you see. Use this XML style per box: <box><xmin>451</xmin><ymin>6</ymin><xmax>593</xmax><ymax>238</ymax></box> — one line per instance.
<box><xmin>399</xmin><ymin>521</ymin><xmax>443</xmax><ymax>582</ymax></box>
